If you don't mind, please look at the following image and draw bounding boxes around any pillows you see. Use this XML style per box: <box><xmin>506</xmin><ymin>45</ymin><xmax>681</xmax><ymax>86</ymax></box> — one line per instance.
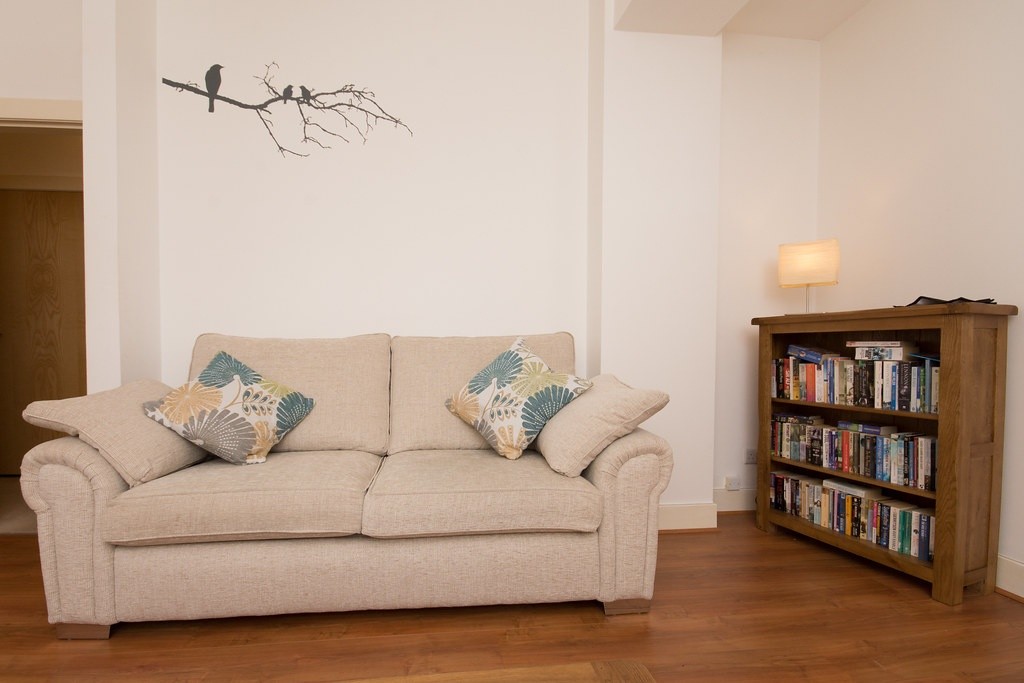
<box><xmin>537</xmin><ymin>370</ymin><xmax>671</xmax><ymax>479</ymax></box>
<box><xmin>141</xmin><ymin>349</ymin><xmax>317</xmax><ymax>463</ymax></box>
<box><xmin>23</xmin><ymin>376</ymin><xmax>207</xmax><ymax>484</ymax></box>
<box><xmin>442</xmin><ymin>336</ymin><xmax>595</xmax><ymax>461</ymax></box>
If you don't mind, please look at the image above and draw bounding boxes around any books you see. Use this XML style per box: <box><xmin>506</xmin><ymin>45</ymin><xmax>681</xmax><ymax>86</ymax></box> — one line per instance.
<box><xmin>766</xmin><ymin>471</ymin><xmax>936</xmax><ymax>563</ymax></box>
<box><xmin>768</xmin><ymin>412</ymin><xmax>938</xmax><ymax>493</ymax></box>
<box><xmin>769</xmin><ymin>340</ymin><xmax>941</xmax><ymax>415</ymax></box>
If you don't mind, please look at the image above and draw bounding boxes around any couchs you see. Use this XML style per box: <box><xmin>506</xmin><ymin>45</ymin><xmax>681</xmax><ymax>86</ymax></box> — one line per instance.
<box><xmin>23</xmin><ymin>332</ymin><xmax>677</xmax><ymax>639</ymax></box>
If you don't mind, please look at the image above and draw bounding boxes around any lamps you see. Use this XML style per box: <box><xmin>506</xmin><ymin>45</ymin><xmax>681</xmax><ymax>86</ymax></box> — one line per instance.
<box><xmin>776</xmin><ymin>238</ymin><xmax>841</xmax><ymax>313</ymax></box>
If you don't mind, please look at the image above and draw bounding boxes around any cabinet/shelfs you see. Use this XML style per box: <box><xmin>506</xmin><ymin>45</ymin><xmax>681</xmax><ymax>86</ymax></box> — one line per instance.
<box><xmin>750</xmin><ymin>303</ymin><xmax>1020</xmax><ymax>605</ymax></box>
